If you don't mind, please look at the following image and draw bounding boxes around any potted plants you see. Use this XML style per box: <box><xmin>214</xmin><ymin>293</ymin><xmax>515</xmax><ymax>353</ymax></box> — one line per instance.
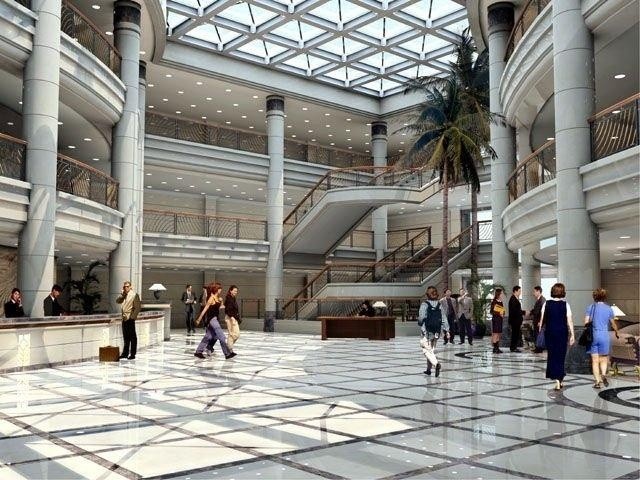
<box><xmin>463</xmin><ymin>262</ymin><xmax>509</xmax><ymax>340</ymax></box>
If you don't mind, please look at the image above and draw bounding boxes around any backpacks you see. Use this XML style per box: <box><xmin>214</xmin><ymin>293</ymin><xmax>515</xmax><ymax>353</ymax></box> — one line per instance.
<box><xmin>425</xmin><ymin>300</ymin><xmax>443</xmax><ymax>334</ymax></box>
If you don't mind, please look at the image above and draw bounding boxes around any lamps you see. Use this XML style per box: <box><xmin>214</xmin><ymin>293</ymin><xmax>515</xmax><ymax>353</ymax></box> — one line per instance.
<box><xmin>371</xmin><ymin>299</ymin><xmax>387</xmax><ymax>315</ymax></box>
<box><xmin>147</xmin><ymin>283</ymin><xmax>168</xmax><ymax>299</ymax></box>
<box><xmin>609</xmin><ymin>304</ymin><xmax>627</xmax><ymax>321</ymax></box>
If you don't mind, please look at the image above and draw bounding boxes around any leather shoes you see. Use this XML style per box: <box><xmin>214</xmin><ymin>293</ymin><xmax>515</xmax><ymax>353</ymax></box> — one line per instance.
<box><xmin>194</xmin><ymin>353</ymin><xmax>206</xmax><ymax>359</ymax></box>
<box><xmin>435</xmin><ymin>363</ymin><xmax>441</xmax><ymax>377</ymax></box>
<box><xmin>511</xmin><ymin>349</ymin><xmax>521</xmax><ymax>353</ymax></box>
<box><xmin>592</xmin><ymin>384</ymin><xmax>600</xmax><ymax>388</ymax></box>
<box><xmin>424</xmin><ymin>371</ymin><xmax>431</xmax><ymax>375</ymax></box>
<box><xmin>225</xmin><ymin>353</ymin><xmax>237</xmax><ymax>359</ymax></box>
<box><xmin>602</xmin><ymin>375</ymin><xmax>609</xmax><ymax>387</ymax></box>
<box><xmin>443</xmin><ymin>340</ymin><xmax>474</xmax><ymax>346</ymax></box>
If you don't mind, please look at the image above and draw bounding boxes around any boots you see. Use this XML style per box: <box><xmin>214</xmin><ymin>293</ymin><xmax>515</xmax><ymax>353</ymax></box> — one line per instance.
<box><xmin>493</xmin><ymin>341</ymin><xmax>503</xmax><ymax>353</ymax></box>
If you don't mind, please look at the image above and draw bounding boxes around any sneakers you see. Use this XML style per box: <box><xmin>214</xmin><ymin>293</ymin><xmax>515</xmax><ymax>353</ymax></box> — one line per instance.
<box><xmin>205</xmin><ymin>349</ymin><xmax>211</xmax><ymax>357</ymax></box>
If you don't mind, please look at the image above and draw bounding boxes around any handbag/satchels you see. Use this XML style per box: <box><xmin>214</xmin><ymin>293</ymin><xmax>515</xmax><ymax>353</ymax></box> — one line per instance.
<box><xmin>578</xmin><ymin>321</ymin><xmax>594</xmax><ymax>347</ymax></box>
<box><xmin>535</xmin><ymin>324</ymin><xmax>548</xmax><ymax>350</ymax></box>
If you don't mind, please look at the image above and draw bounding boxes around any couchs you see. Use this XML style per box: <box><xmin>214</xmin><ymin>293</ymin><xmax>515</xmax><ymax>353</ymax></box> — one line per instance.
<box><xmin>605</xmin><ymin>322</ymin><xmax>640</xmax><ymax>377</ymax></box>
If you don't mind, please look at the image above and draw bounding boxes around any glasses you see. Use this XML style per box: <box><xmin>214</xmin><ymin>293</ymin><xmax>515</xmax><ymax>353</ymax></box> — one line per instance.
<box><xmin>124</xmin><ymin>286</ymin><xmax>130</xmax><ymax>288</ymax></box>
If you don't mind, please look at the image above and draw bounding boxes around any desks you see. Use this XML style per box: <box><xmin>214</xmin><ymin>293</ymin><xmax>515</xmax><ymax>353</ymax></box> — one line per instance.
<box><xmin>316</xmin><ymin>315</ymin><xmax>397</xmax><ymax>341</ymax></box>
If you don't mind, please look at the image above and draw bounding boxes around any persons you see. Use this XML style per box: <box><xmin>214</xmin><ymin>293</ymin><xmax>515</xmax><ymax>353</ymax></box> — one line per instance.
<box><xmin>508</xmin><ymin>286</ymin><xmax>529</xmax><ymax>352</ymax></box>
<box><xmin>182</xmin><ymin>281</ymin><xmax>241</xmax><ymax>359</ymax></box>
<box><xmin>490</xmin><ymin>288</ymin><xmax>505</xmax><ymax>353</ymax></box>
<box><xmin>418</xmin><ymin>286</ymin><xmax>451</xmax><ymax>377</ymax></box>
<box><xmin>356</xmin><ymin>301</ymin><xmax>374</xmax><ymax>317</ymax></box>
<box><xmin>439</xmin><ymin>289</ymin><xmax>457</xmax><ymax>345</ymax></box>
<box><xmin>43</xmin><ymin>285</ymin><xmax>66</xmax><ymax>316</ymax></box>
<box><xmin>457</xmin><ymin>288</ymin><xmax>473</xmax><ymax>345</ymax></box>
<box><xmin>578</xmin><ymin>389</ymin><xmax>621</xmax><ymax>480</ymax></box>
<box><xmin>184</xmin><ymin>335</ymin><xmax>239</xmax><ymax>392</ymax></box>
<box><xmin>121</xmin><ymin>359</ymin><xmax>137</xmax><ymax>400</ymax></box>
<box><xmin>420</xmin><ymin>374</ymin><xmax>441</xmax><ymax>421</ymax></box>
<box><xmin>535</xmin><ymin>391</ymin><xmax>575</xmax><ymax>480</ymax></box>
<box><xmin>538</xmin><ymin>283</ymin><xmax>575</xmax><ymax>391</ymax></box>
<box><xmin>115</xmin><ymin>282</ymin><xmax>140</xmax><ymax>359</ymax></box>
<box><xmin>530</xmin><ymin>286</ymin><xmax>546</xmax><ymax>353</ymax></box>
<box><xmin>584</xmin><ymin>288</ymin><xmax>620</xmax><ymax>388</ymax></box>
<box><xmin>4</xmin><ymin>288</ymin><xmax>24</xmax><ymax>318</ymax></box>
